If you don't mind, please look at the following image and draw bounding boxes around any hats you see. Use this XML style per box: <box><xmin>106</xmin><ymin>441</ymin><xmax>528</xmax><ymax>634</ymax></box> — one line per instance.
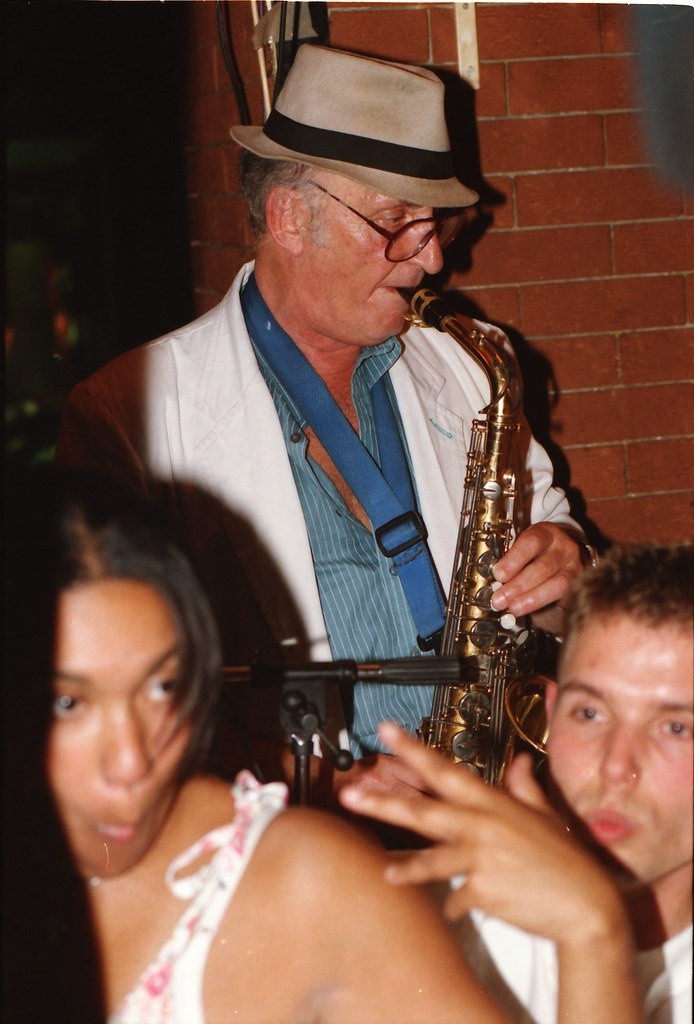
<box><xmin>229</xmin><ymin>42</ymin><xmax>479</xmax><ymax>209</ymax></box>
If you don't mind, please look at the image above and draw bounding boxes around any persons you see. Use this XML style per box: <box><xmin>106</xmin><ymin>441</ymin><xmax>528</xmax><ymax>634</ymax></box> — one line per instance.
<box><xmin>431</xmin><ymin>535</ymin><xmax>693</xmax><ymax>1024</ymax></box>
<box><xmin>57</xmin><ymin>42</ymin><xmax>622</xmax><ymax>850</ymax></box>
<box><xmin>3</xmin><ymin>477</ymin><xmax>644</xmax><ymax>1024</ymax></box>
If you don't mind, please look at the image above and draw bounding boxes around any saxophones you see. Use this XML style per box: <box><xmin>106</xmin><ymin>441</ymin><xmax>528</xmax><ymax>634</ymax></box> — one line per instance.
<box><xmin>396</xmin><ymin>283</ymin><xmax>559</xmax><ymax>793</ymax></box>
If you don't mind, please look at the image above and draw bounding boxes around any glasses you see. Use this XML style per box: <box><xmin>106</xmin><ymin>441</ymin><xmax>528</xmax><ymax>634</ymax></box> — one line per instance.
<box><xmin>306</xmin><ymin>178</ymin><xmax>468</xmax><ymax>262</ymax></box>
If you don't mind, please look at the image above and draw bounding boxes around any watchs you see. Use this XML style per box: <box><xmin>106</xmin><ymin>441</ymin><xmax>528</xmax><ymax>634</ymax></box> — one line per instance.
<box><xmin>567</xmin><ymin>530</ymin><xmax>602</xmax><ymax>569</ymax></box>
